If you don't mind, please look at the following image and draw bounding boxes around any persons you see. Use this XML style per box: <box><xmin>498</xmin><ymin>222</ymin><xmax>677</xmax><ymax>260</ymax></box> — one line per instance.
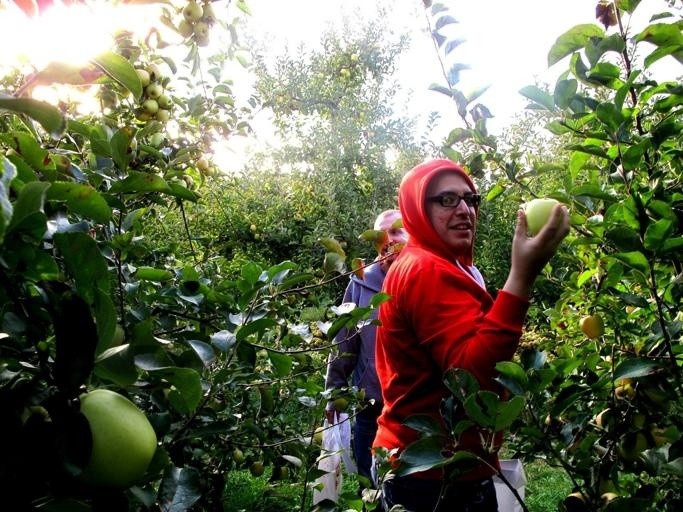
<box><xmin>319</xmin><ymin>209</ymin><xmax>409</xmax><ymax>511</ymax></box>
<box><xmin>368</xmin><ymin>158</ymin><xmax>570</xmax><ymax>510</ymax></box>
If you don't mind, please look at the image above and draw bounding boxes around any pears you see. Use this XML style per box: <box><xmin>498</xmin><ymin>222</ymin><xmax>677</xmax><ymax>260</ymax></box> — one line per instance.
<box><xmin>68</xmin><ymin>1</ymin><xmax>639</xmax><ymax>512</ymax></box>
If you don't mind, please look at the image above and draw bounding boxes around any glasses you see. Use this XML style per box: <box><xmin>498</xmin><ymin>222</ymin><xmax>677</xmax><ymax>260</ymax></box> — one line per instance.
<box><xmin>426</xmin><ymin>194</ymin><xmax>481</xmax><ymax>207</ymax></box>
